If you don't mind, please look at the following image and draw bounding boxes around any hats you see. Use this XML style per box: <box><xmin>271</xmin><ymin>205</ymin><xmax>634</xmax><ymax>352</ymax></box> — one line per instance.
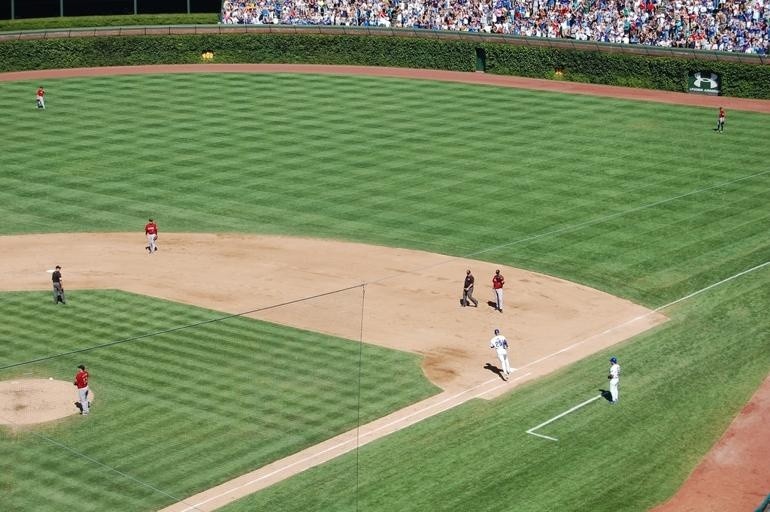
<box><xmin>610</xmin><ymin>358</ymin><xmax>617</xmax><ymax>363</ymax></box>
<box><xmin>495</xmin><ymin>329</ymin><xmax>499</xmax><ymax>334</ymax></box>
<box><xmin>77</xmin><ymin>364</ymin><xmax>85</xmax><ymax>371</ymax></box>
<box><xmin>55</xmin><ymin>265</ymin><xmax>62</xmax><ymax>269</ymax></box>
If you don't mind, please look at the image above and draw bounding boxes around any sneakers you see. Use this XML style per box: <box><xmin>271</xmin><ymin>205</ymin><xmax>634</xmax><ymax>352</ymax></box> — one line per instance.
<box><xmin>460</xmin><ymin>302</ymin><xmax>479</xmax><ymax>307</ymax></box>
<box><xmin>609</xmin><ymin>399</ymin><xmax>618</xmax><ymax>404</ymax></box>
<box><xmin>495</xmin><ymin>306</ymin><xmax>503</xmax><ymax>313</ymax></box>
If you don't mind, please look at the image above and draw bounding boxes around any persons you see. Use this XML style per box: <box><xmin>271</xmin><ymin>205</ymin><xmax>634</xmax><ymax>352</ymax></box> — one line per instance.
<box><xmin>36</xmin><ymin>86</ymin><xmax>45</xmax><ymax>109</ymax></box>
<box><xmin>462</xmin><ymin>269</ymin><xmax>478</xmax><ymax>307</ymax></box>
<box><xmin>492</xmin><ymin>270</ymin><xmax>505</xmax><ymax>313</ymax></box>
<box><xmin>714</xmin><ymin>107</ymin><xmax>726</xmax><ymax>133</ymax></box>
<box><xmin>489</xmin><ymin>329</ymin><xmax>512</xmax><ymax>376</ymax></box>
<box><xmin>608</xmin><ymin>357</ymin><xmax>621</xmax><ymax>404</ymax></box>
<box><xmin>73</xmin><ymin>365</ymin><xmax>90</xmax><ymax>415</ymax></box>
<box><xmin>52</xmin><ymin>265</ymin><xmax>65</xmax><ymax>304</ymax></box>
<box><xmin>144</xmin><ymin>218</ymin><xmax>158</xmax><ymax>254</ymax></box>
<box><xmin>221</xmin><ymin>0</ymin><xmax>769</xmax><ymax>55</ymax></box>
